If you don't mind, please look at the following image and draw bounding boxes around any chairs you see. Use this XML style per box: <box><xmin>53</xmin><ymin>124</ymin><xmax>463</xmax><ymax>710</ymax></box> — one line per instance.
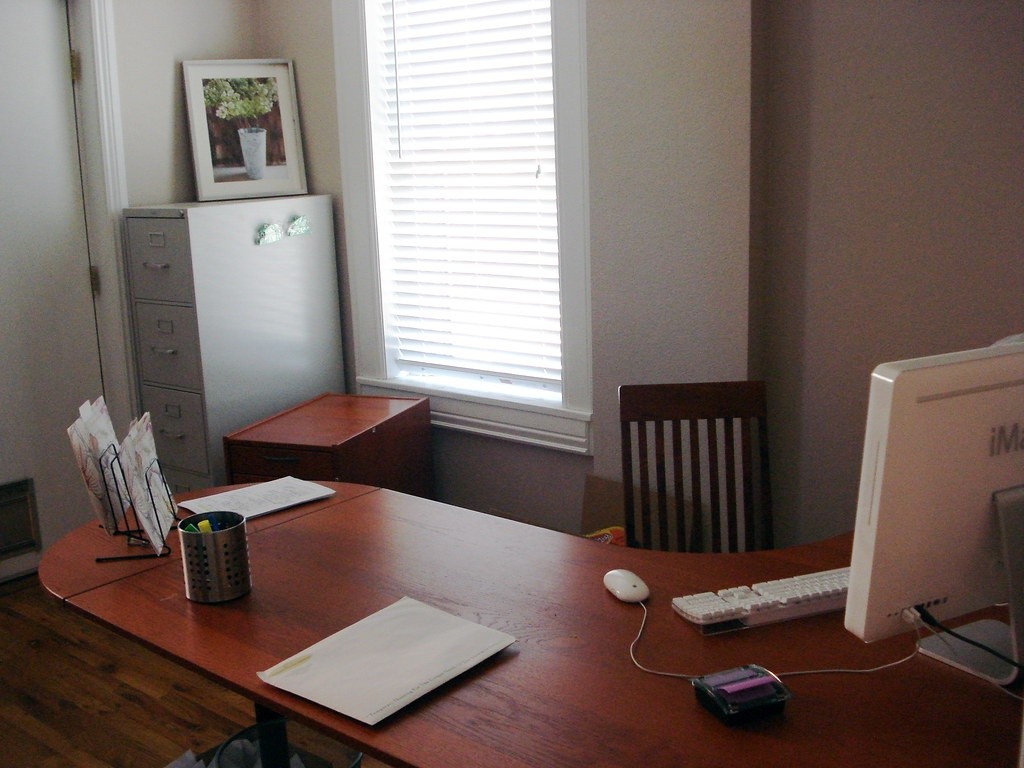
<box><xmin>618</xmin><ymin>380</ymin><xmax>775</xmax><ymax>555</ymax></box>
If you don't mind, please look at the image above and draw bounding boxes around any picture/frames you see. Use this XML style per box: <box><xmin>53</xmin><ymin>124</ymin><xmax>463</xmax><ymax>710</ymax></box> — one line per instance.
<box><xmin>180</xmin><ymin>58</ymin><xmax>309</xmax><ymax>203</ymax></box>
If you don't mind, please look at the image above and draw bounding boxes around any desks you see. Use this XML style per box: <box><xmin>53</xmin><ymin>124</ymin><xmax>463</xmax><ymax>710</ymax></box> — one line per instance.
<box><xmin>36</xmin><ymin>479</ymin><xmax>1024</xmax><ymax>768</ymax></box>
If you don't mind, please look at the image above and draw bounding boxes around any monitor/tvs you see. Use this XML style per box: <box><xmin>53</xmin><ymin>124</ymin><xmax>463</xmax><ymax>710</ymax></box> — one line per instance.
<box><xmin>845</xmin><ymin>346</ymin><xmax>1024</xmax><ymax>687</ymax></box>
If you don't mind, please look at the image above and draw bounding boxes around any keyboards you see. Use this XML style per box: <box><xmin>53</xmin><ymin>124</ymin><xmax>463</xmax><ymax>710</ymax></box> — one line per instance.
<box><xmin>670</xmin><ymin>566</ymin><xmax>853</xmax><ymax>636</ymax></box>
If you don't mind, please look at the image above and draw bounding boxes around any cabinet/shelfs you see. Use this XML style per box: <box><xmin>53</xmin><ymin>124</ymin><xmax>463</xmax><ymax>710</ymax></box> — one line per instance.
<box><xmin>217</xmin><ymin>390</ymin><xmax>435</xmax><ymax>502</ymax></box>
<box><xmin>121</xmin><ymin>195</ymin><xmax>345</xmax><ymax>495</ymax></box>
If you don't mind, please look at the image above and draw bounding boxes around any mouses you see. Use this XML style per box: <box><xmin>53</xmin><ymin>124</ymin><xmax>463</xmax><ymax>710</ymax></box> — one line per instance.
<box><xmin>604</xmin><ymin>569</ymin><xmax>650</xmax><ymax>602</ymax></box>
